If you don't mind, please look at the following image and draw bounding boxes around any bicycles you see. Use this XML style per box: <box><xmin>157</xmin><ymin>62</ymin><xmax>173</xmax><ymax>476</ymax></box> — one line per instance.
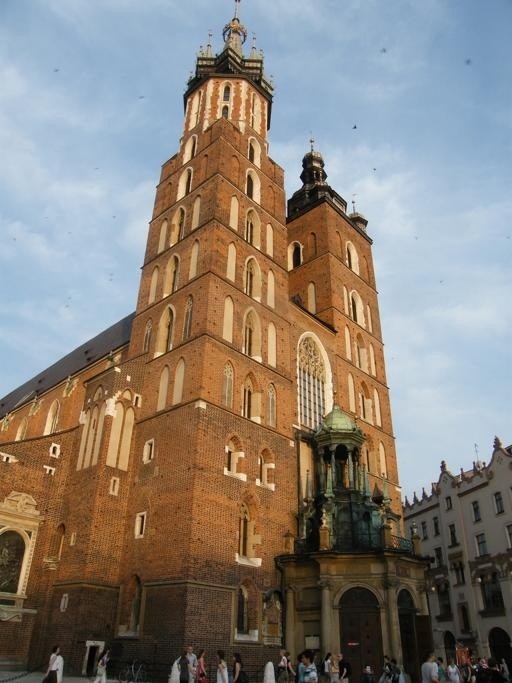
<box><xmin>118</xmin><ymin>658</ymin><xmax>149</xmax><ymax>682</ymax></box>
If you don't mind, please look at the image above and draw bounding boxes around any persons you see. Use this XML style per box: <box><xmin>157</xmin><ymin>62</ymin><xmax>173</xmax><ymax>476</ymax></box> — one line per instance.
<box><xmin>42</xmin><ymin>646</ymin><xmax>60</xmax><ymax>683</ymax></box>
<box><xmin>94</xmin><ymin>647</ymin><xmax>110</xmax><ymax>683</ymax></box>
